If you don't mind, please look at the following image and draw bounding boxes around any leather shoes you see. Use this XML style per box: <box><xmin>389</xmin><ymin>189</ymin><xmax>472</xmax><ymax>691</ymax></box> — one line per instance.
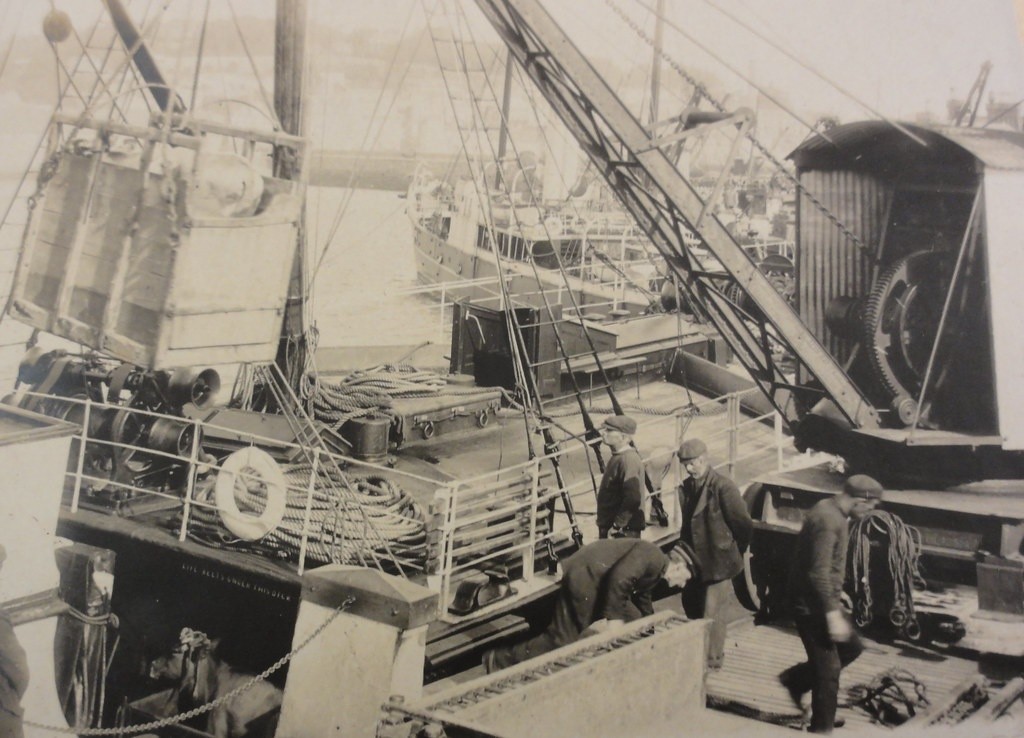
<box><xmin>482</xmin><ymin>651</ymin><xmax>497</xmax><ymax>673</ymax></box>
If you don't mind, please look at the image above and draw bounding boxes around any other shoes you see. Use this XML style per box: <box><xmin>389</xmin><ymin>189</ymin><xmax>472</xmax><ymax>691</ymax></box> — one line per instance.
<box><xmin>779</xmin><ymin>669</ymin><xmax>808</xmax><ymax>713</ymax></box>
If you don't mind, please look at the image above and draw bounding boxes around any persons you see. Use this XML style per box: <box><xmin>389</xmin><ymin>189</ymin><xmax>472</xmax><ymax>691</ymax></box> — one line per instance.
<box><xmin>483</xmin><ymin>538</ymin><xmax>703</xmax><ymax>677</ymax></box>
<box><xmin>675</xmin><ymin>439</ymin><xmax>752</xmax><ymax>672</ymax></box>
<box><xmin>595</xmin><ymin>415</ymin><xmax>645</xmax><ymax>538</ymax></box>
<box><xmin>778</xmin><ymin>474</ymin><xmax>884</xmax><ymax>736</ymax></box>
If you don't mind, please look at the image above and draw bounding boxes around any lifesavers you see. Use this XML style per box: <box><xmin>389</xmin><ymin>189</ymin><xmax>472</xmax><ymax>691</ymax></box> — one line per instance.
<box><xmin>187</xmin><ymin>150</ymin><xmax>264</xmax><ymax>216</ymax></box>
<box><xmin>213</xmin><ymin>443</ymin><xmax>289</xmax><ymax>543</ymax></box>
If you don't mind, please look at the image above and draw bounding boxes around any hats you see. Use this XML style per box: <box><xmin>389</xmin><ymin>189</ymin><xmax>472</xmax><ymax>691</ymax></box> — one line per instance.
<box><xmin>843</xmin><ymin>474</ymin><xmax>884</xmax><ymax>499</ymax></box>
<box><xmin>673</xmin><ymin>539</ymin><xmax>703</xmax><ymax>578</ymax></box>
<box><xmin>677</xmin><ymin>438</ymin><xmax>708</xmax><ymax>464</ymax></box>
<box><xmin>603</xmin><ymin>415</ymin><xmax>637</xmax><ymax>436</ymax></box>
